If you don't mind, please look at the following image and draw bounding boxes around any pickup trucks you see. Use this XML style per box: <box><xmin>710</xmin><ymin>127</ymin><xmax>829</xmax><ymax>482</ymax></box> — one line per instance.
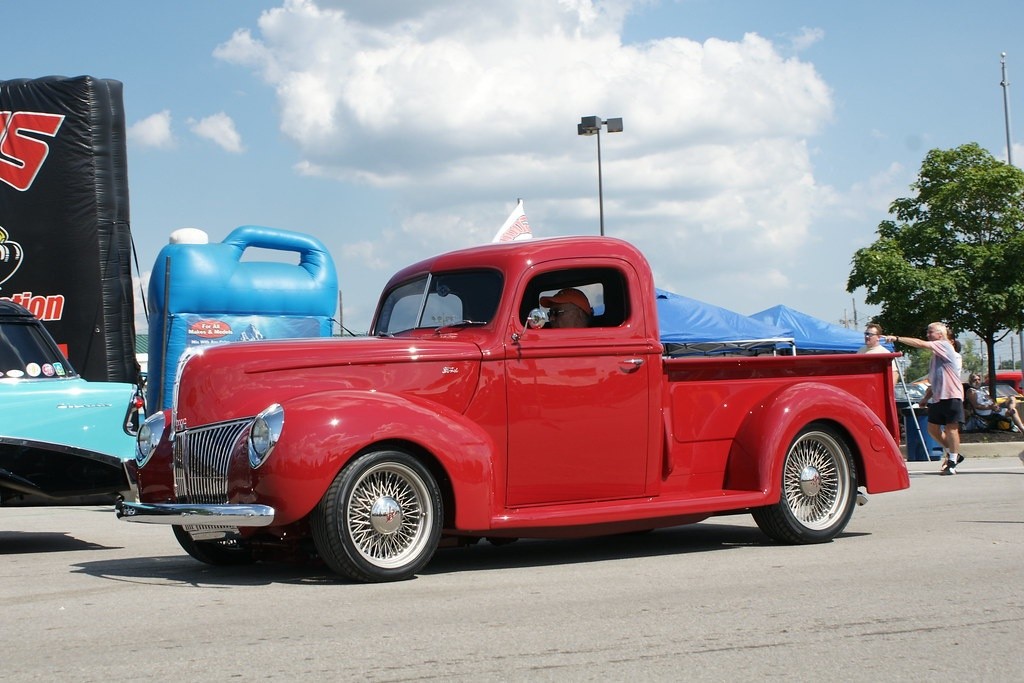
<box><xmin>113</xmin><ymin>236</ymin><xmax>911</xmax><ymax>582</ymax></box>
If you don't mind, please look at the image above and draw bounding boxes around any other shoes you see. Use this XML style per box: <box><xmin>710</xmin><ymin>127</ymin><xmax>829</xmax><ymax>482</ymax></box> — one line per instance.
<box><xmin>942</xmin><ymin>453</ymin><xmax>964</xmax><ymax>468</ymax></box>
<box><xmin>944</xmin><ymin>459</ymin><xmax>956</xmax><ymax>474</ymax></box>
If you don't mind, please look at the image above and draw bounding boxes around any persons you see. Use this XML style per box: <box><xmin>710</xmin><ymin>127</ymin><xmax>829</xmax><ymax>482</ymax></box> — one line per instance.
<box><xmin>540</xmin><ymin>289</ymin><xmax>594</xmax><ymax>327</ymax></box>
<box><xmin>882</xmin><ymin>322</ymin><xmax>964</xmax><ymax>475</ymax></box>
<box><xmin>856</xmin><ymin>323</ymin><xmax>899</xmax><ymax>390</ymax></box>
<box><xmin>967</xmin><ymin>374</ymin><xmax>1024</xmax><ymax>432</ymax></box>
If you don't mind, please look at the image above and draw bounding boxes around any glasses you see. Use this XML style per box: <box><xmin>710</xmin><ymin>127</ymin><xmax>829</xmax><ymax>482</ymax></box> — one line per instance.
<box><xmin>864</xmin><ymin>332</ymin><xmax>879</xmax><ymax>337</ymax></box>
<box><xmin>547</xmin><ymin>308</ymin><xmax>578</xmax><ymax>318</ymax></box>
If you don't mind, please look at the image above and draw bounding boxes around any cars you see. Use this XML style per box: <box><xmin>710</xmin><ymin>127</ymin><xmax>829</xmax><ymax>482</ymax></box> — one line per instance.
<box><xmin>981</xmin><ymin>369</ymin><xmax>1024</xmax><ymax>408</ymax></box>
<box><xmin>894</xmin><ymin>383</ymin><xmax>932</xmax><ymax>425</ymax></box>
<box><xmin>0</xmin><ymin>298</ymin><xmax>147</xmax><ymax>506</ymax></box>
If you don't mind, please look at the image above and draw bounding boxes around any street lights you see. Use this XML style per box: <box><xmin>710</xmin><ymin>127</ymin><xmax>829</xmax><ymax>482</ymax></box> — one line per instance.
<box><xmin>577</xmin><ymin>116</ymin><xmax>624</xmax><ymax>235</ymax></box>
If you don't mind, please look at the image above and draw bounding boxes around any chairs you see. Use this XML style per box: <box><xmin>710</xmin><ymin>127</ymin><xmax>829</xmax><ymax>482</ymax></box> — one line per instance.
<box><xmin>960</xmin><ymin>398</ymin><xmax>996</xmax><ymax>433</ymax></box>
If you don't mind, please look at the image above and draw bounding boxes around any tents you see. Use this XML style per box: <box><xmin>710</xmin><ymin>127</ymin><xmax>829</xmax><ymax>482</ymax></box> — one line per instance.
<box><xmin>597</xmin><ymin>289</ymin><xmax>797</xmax><ymax>356</ymax></box>
<box><xmin>749</xmin><ymin>305</ymin><xmax>931</xmax><ymax>462</ymax></box>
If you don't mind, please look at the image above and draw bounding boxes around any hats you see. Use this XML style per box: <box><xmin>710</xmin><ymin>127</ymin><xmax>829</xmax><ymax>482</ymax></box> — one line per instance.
<box><xmin>540</xmin><ymin>287</ymin><xmax>592</xmax><ymax>317</ymax></box>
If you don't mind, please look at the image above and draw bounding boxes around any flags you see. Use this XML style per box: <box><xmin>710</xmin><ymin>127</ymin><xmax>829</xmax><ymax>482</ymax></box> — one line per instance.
<box><xmin>493</xmin><ymin>200</ymin><xmax>532</xmax><ymax>244</ymax></box>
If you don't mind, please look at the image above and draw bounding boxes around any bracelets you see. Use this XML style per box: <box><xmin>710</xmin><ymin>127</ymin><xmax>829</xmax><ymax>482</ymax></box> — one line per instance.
<box><xmin>897</xmin><ymin>336</ymin><xmax>898</xmax><ymax>341</ymax></box>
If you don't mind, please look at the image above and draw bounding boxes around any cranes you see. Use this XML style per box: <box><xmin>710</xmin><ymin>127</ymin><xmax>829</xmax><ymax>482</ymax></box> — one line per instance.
<box><xmin>839</xmin><ymin>298</ymin><xmax>871</xmax><ymax>331</ymax></box>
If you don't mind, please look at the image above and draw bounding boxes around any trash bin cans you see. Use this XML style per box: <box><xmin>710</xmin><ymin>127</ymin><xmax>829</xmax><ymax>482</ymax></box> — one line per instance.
<box><xmin>902</xmin><ymin>408</ymin><xmax>946</xmax><ymax>462</ymax></box>
